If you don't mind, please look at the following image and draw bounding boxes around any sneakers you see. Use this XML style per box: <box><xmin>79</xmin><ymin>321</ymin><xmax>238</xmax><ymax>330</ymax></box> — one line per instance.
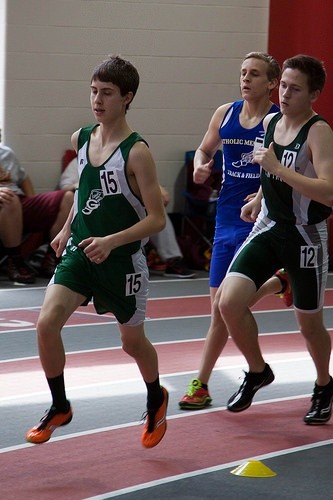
<box><xmin>143</xmin><ymin>387</ymin><xmax>168</xmax><ymax>448</ymax></box>
<box><xmin>29</xmin><ymin>400</ymin><xmax>72</xmax><ymax>443</ymax></box>
<box><xmin>275</xmin><ymin>267</ymin><xmax>293</xmax><ymax>307</ymax></box>
<box><xmin>228</xmin><ymin>363</ymin><xmax>274</xmax><ymax>412</ymax></box>
<box><xmin>163</xmin><ymin>260</ymin><xmax>196</xmax><ymax>278</ymax></box>
<box><xmin>7</xmin><ymin>256</ymin><xmax>36</xmax><ymax>284</ymax></box>
<box><xmin>304</xmin><ymin>376</ymin><xmax>333</xmax><ymax>425</ymax></box>
<box><xmin>180</xmin><ymin>380</ymin><xmax>211</xmax><ymax>409</ymax></box>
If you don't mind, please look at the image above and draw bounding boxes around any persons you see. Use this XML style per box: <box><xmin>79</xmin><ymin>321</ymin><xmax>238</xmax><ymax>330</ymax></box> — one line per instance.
<box><xmin>0</xmin><ymin>126</ymin><xmax>226</xmax><ymax>284</ymax></box>
<box><xmin>218</xmin><ymin>54</ymin><xmax>333</xmax><ymax>426</ymax></box>
<box><xmin>178</xmin><ymin>51</ymin><xmax>294</xmax><ymax>410</ymax></box>
<box><xmin>25</xmin><ymin>55</ymin><xmax>169</xmax><ymax>448</ymax></box>
